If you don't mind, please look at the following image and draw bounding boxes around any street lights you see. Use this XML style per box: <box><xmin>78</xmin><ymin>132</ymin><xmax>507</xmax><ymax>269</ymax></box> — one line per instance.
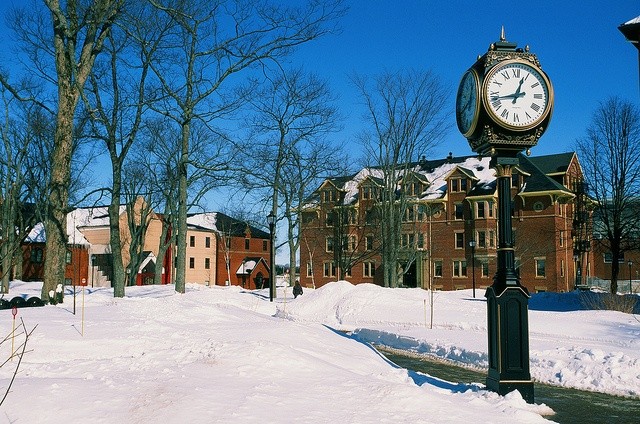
<box><xmin>469</xmin><ymin>238</ymin><xmax>477</xmax><ymax>297</ymax></box>
<box><xmin>242</xmin><ymin>259</ymin><xmax>245</xmax><ymax>287</ymax></box>
<box><xmin>267</xmin><ymin>210</ymin><xmax>277</xmax><ymax>301</ymax></box>
<box><xmin>627</xmin><ymin>260</ymin><xmax>633</xmax><ymax>294</ymax></box>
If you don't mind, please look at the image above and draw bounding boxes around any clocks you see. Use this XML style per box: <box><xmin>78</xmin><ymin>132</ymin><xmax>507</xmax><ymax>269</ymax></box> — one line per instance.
<box><xmin>456</xmin><ymin>68</ymin><xmax>481</xmax><ymax>137</ymax></box>
<box><xmin>481</xmin><ymin>58</ymin><xmax>554</xmax><ymax>131</ymax></box>
